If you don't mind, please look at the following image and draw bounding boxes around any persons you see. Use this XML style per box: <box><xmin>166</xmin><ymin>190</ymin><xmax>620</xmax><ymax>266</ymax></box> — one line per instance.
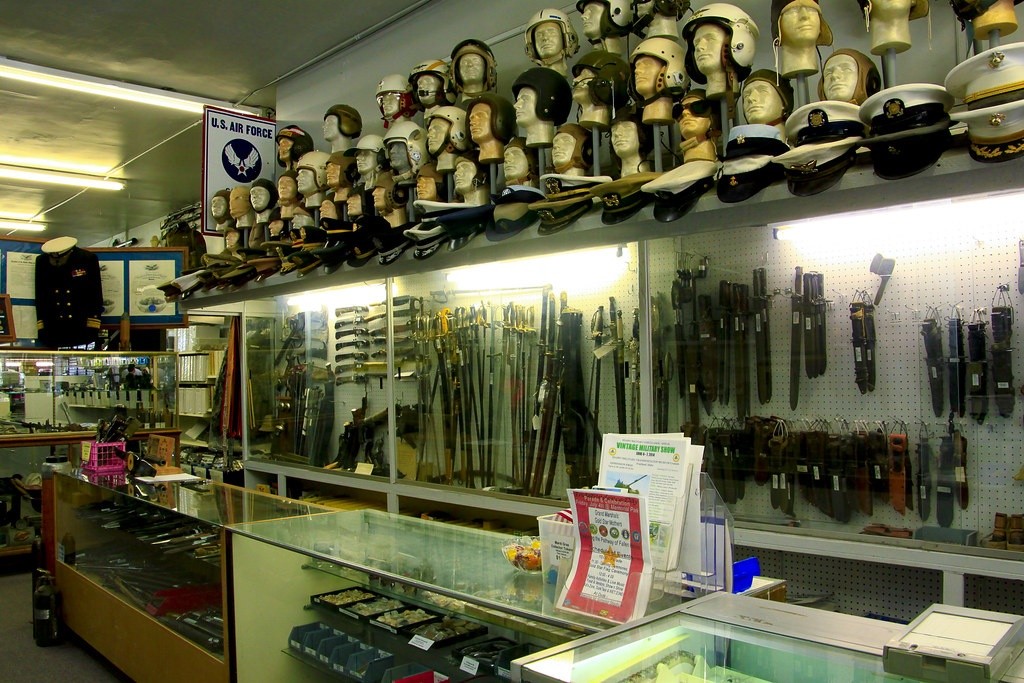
<box><xmin>119</xmin><ymin>363</ymin><xmax>152</xmax><ymax>390</ymax></box>
<box><xmin>211</xmin><ymin>0</ymin><xmax>1024</xmax><ymax>254</ymax></box>
<box><xmin>34</xmin><ymin>236</ymin><xmax>103</xmax><ymax>347</ymax></box>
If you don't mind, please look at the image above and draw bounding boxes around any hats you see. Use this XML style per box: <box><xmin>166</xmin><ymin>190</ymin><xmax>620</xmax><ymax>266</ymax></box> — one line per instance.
<box><xmin>157</xmin><ymin>0</ymin><xmax>1024</xmax><ymax>303</ymax></box>
<box><xmin>41</xmin><ymin>236</ymin><xmax>78</xmax><ymax>253</ymax></box>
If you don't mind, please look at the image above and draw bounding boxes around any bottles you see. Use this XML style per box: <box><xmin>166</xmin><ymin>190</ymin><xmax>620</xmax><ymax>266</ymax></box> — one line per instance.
<box><xmin>63</xmin><ymin>530</ymin><xmax>76</xmax><ymax>565</ymax></box>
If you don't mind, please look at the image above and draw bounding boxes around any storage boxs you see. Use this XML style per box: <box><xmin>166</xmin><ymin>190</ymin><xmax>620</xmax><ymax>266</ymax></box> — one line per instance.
<box><xmin>537</xmin><ymin>514</ymin><xmax>575</xmax><ymax>584</ymax></box>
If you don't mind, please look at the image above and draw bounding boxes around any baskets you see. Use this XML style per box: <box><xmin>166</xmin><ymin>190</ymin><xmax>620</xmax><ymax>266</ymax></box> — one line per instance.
<box><xmin>82</xmin><ymin>442</ymin><xmax>126</xmax><ymax>472</ymax></box>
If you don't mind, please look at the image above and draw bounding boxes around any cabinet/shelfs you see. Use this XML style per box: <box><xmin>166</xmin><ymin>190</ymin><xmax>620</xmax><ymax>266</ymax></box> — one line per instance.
<box><xmin>228</xmin><ymin>510</ymin><xmax>687</xmax><ymax>683</ymax></box>
<box><xmin>54</xmin><ymin>469</ymin><xmax>345</xmax><ymax>683</ymax></box>
<box><xmin>175</xmin><ymin>350</ymin><xmax>226</xmax><ymax>447</ymax></box>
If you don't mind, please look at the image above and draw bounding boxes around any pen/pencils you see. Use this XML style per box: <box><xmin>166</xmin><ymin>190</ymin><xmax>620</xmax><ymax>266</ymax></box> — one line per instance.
<box><xmin>178</xmin><ymin>480</ymin><xmax>207</xmax><ymax>484</ymax></box>
